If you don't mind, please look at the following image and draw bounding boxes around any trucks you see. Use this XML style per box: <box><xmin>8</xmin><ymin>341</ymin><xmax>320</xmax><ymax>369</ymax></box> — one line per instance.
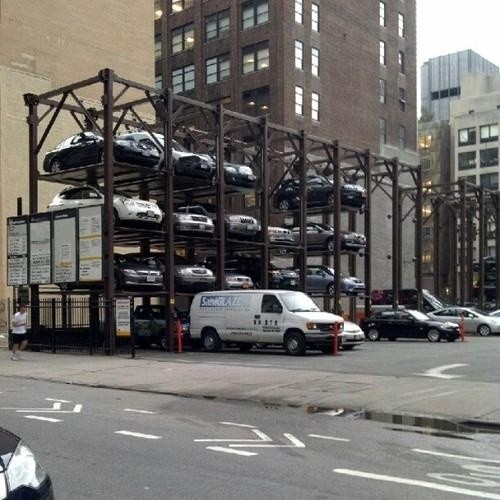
<box><xmin>372</xmin><ymin>288</ymin><xmax>450</xmax><ymax>313</ymax></box>
<box><xmin>189</xmin><ymin>290</ymin><xmax>344</xmax><ymax>355</ymax></box>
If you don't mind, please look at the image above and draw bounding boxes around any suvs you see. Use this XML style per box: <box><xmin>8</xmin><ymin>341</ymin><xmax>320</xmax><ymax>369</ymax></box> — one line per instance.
<box><xmin>125</xmin><ymin>252</ymin><xmax>302</xmax><ymax>289</ymax></box>
<box><xmin>272</xmin><ymin>174</ymin><xmax>367</xmax><ymax>210</ymax></box>
<box><xmin>132</xmin><ymin>303</ymin><xmax>181</xmax><ymax>347</ymax></box>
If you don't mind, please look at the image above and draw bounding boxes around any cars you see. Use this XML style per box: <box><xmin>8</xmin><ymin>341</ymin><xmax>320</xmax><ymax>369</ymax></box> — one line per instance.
<box><xmin>0</xmin><ymin>424</ymin><xmax>58</xmax><ymax>500</ymax></box>
<box><xmin>155</xmin><ymin>204</ymin><xmax>216</xmax><ymax>235</ymax></box>
<box><xmin>150</xmin><ymin>311</ymin><xmax>188</xmax><ymax>352</ymax></box>
<box><xmin>339</xmin><ymin>320</ymin><xmax>365</xmax><ymax>351</ymax></box>
<box><xmin>356</xmin><ymin>308</ymin><xmax>461</xmax><ymax>342</ymax></box>
<box><xmin>201</xmin><ymin>203</ymin><xmax>262</xmax><ymax>241</ymax></box>
<box><xmin>427</xmin><ymin>305</ymin><xmax>500</xmax><ymax>335</ymax></box>
<box><xmin>114</xmin><ymin>130</ymin><xmax>217</xmax><ymax>179</ymax></box>
<box><xmin>46</xmin><ymin>183</ymin><xmax>167</xmax><ymax>229</ymax></box>
<box><xmin>113</xmin><ymin>253</ymin><xmax>165</xmax><ymax>290</ymax></box>
<box><xmin>42</xmin><ymin>129</ymin><xmax>162</xmax><ymax>167</ymax></box>
<box><xmin>197</xmin><ymin>153</ymin><xmax>258</xmax><ymax>189</ymax></box>
<box><xmin>287</xmin><ymin>222</ymin><xmax>368</xmax><ymax>252</ymax></box>
<box><xmin>464</xmin><ymin>254</ymin><xmax>500</xmax><ymax>316</ymax></box>
<box><xmin>268</xmin><ymin>225</ymin><xmax>296</xmax><ymax>245</ymax></box>
<box><xmin>291</xmin><ymin>263</ymin><xmax>368</xmax><ymax>297</ymax></box>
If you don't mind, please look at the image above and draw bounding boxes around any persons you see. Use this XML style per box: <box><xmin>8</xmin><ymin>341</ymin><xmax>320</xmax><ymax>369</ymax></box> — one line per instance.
<box><xmin>8</xmin><ymin>302</ymin><xmax>31</xmax><ymax>362</ymax></box>
<box><xmin>457</xmin><ymin>313</ymin><xmax>466</xmax><ymax>342</ymax></box>
